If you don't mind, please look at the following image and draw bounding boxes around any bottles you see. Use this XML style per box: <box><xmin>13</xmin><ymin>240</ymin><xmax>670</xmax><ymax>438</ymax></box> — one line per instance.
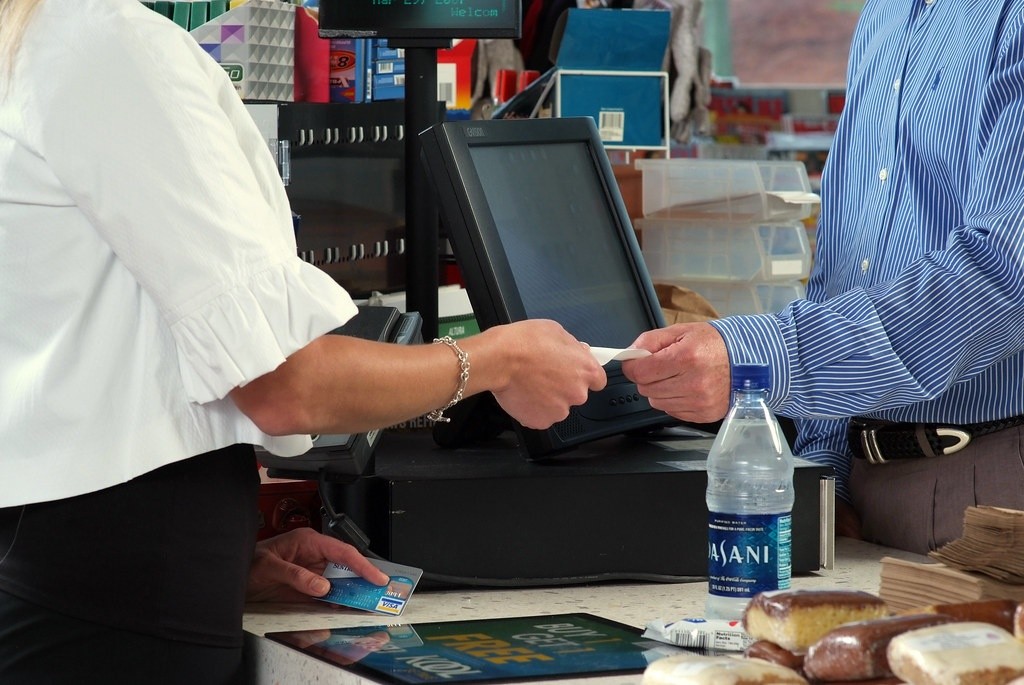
<box><xmin>706</xmin><ymin>365</ymin><xmax>798</xmax><ymax>623</ymax></box>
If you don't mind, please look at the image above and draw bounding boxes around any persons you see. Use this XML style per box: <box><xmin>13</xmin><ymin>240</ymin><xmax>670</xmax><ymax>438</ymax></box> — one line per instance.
<box><xmin>0</xmin><ymin>0</ymin><xmax>607</xmax><ymax>685</ymax></box>
<box><xmin>621</xmin><ymin>0</ymin><xmax>1024</xmax><ymax>557</ymax></box>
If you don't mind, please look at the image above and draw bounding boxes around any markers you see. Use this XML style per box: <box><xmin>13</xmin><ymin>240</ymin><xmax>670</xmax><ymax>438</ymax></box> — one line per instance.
<box><xmin>494</xmin><ymin>69</ymin><xmax>540</xmax><ymax>107</ymax></box>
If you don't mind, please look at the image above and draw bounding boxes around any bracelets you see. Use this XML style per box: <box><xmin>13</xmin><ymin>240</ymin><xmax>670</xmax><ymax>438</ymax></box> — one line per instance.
<box><xmin>426</xmin><ymin>336</ymin><xmax>469</xmax><ymax>422</ymax></box>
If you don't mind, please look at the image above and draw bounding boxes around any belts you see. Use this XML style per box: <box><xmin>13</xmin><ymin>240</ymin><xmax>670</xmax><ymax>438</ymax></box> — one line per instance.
<box><xmin>846</xmin><ymin>415</ymin><xmax>1022</xmax><ymax>464</ymax></box>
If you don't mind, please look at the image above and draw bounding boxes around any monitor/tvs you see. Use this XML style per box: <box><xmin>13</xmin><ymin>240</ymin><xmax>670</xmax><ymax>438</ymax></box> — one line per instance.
<box><xmin>418</xmin><ymin>116</ymin><xmax>680</xmax><ymax>466</ymax></box>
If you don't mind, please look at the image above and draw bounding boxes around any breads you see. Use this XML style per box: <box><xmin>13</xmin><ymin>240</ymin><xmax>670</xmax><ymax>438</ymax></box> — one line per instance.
<box><xmin>641</xmin><ymin>585</ymin><xmax>1024</xmax><ymax>685</ymax></box>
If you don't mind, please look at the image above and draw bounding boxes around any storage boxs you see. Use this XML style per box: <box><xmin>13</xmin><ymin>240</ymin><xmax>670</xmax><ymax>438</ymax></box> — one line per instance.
<box><xmin>635</xmin><ymin>159</ymin><xmax>811</xmax><ymax>322</ymax></box>
